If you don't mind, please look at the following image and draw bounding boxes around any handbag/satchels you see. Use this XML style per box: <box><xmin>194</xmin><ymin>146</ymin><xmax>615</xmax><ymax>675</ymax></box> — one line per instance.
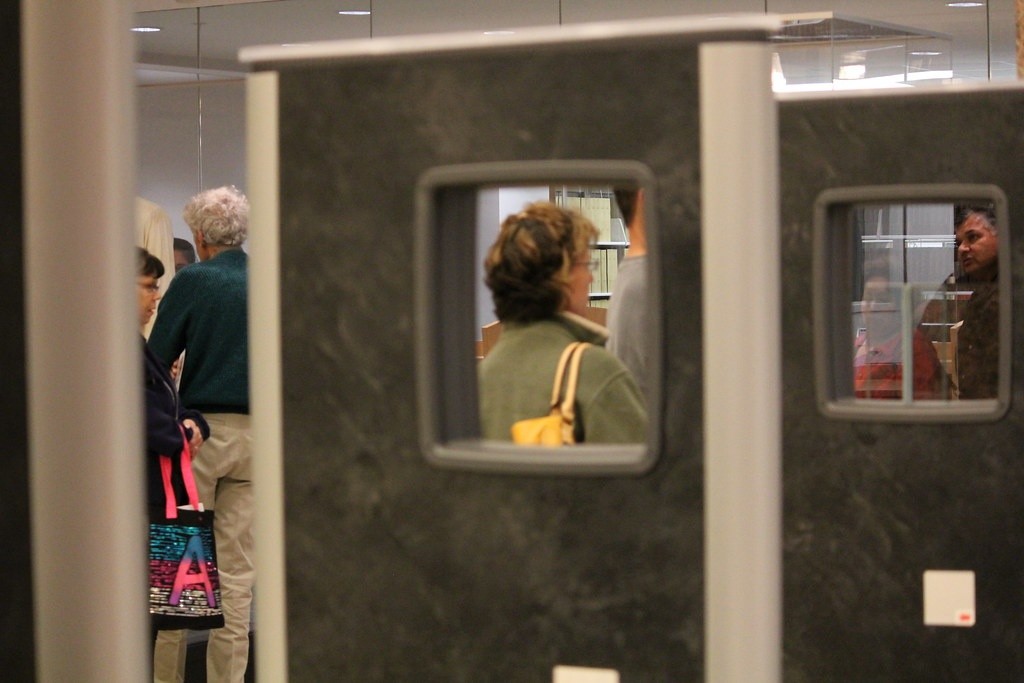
<box><xmin>149</xmin><ymin>423</ymin><xmax>224</xmax><ymax>630</ymax></box>
<box><xmin>511</xmin><ymin>341</ymin><xmax>591</xmax><ymax>449</ymax></box>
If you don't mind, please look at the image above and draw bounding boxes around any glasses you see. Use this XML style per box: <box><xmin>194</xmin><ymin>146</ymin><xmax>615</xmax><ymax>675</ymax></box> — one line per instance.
<box><xmin>139</xmin><ymin>283</ymin><xmax>159</xmax><ymax>293</ymax></box>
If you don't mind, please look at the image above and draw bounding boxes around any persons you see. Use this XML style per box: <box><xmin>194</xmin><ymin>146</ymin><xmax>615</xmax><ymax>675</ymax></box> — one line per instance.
<box><xmin>133</xmin><ymin>187</ymin><xmax>258</xmax><ymax>682</ymax></box>
<box><xmin>476</xmin><ymin>182</ymin><xmax>648</xmax><ymax>446</ymax></box>
<box><xmin>853</xmin><ymin>201</ymin><xmax>1001</xmax><ymax>401</ymax></box>
